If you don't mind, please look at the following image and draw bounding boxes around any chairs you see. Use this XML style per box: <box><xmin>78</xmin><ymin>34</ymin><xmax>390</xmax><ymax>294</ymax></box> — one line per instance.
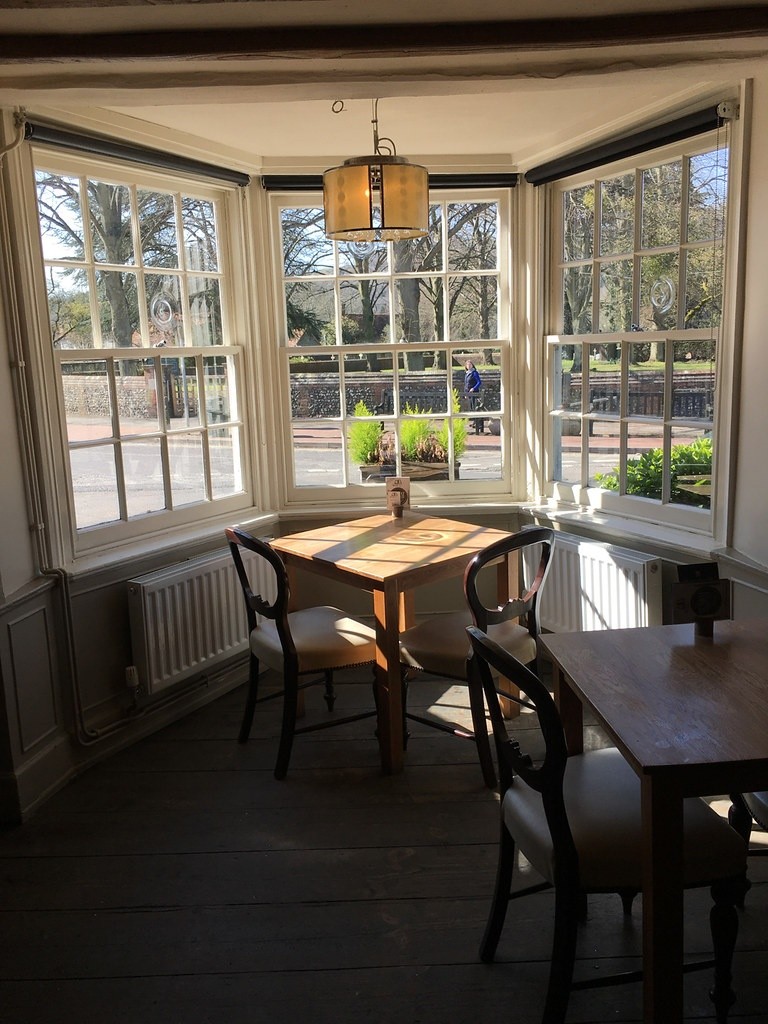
<box><xmin>463</xmin><ymin>624</ymin><xmax>753</xmax><ymax>1024</ymax></box>
<box><xmin>223</xmin><ymin>528</ymin><xmax>424</xmax><ymax>781</ymax></box>
<box><xmin>400</xmin><ymin>525</ymin><xmax>556</xmax><ymax>754</ymax></box>
<box><xmin>727</xmin><ymin>791</ymin><xmax>768</xmax><ymax>912</ymax></box>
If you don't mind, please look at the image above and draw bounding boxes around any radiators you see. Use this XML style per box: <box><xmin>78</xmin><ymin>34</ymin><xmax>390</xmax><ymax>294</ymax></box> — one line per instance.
<box><xmin>520</xmin><ymin>524</ymin><xmax>663</xmax><ymax>633</ymax></box>
<box><xmin>124</xmin><ymin>536</ymin><xmax>279</xmax><ymax>704</ymax></box>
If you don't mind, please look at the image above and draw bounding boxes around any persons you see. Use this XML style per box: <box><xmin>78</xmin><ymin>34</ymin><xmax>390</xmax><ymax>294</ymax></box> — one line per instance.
<box><xmin>464</xmin><ymin>359</ymin><xmax>482</xmax><ymax>421</ymax></box>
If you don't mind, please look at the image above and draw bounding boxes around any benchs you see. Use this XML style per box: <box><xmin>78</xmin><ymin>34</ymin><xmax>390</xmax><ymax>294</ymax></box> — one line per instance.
<box><xmin>589</xmin><ymin>391</ymin><xmax>712</xmax><ymax>437</ymax></box>
<box><xmin>373</xmin><ymin>389</ymin><xmax>447</xmax><ymax>416</ymax></box>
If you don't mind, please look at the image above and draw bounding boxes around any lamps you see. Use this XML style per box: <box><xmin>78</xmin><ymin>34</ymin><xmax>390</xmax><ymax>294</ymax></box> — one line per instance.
<box><xmin>322</xmin><ymin>98</ymin><xmax>430</xmax><ymax>243</ymax></box>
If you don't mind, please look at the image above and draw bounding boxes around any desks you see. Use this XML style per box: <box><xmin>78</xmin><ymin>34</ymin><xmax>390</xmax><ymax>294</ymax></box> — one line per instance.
<box><xmin>537</xmin><ymin>619</ymin><xmax>768</xmax><ymax>1024</ymax></box>
<box><xmin>268</xmin><ymin>512</ymin><xmax>520</xmax><ymax>777</ymax></box>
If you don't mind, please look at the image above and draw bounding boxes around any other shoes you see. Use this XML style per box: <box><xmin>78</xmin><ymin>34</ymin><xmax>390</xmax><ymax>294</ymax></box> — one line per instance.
<box><xmin>469</xmin><ymin>423</ymin><xmax>483</xmax><ymax>429</ymax></box>
